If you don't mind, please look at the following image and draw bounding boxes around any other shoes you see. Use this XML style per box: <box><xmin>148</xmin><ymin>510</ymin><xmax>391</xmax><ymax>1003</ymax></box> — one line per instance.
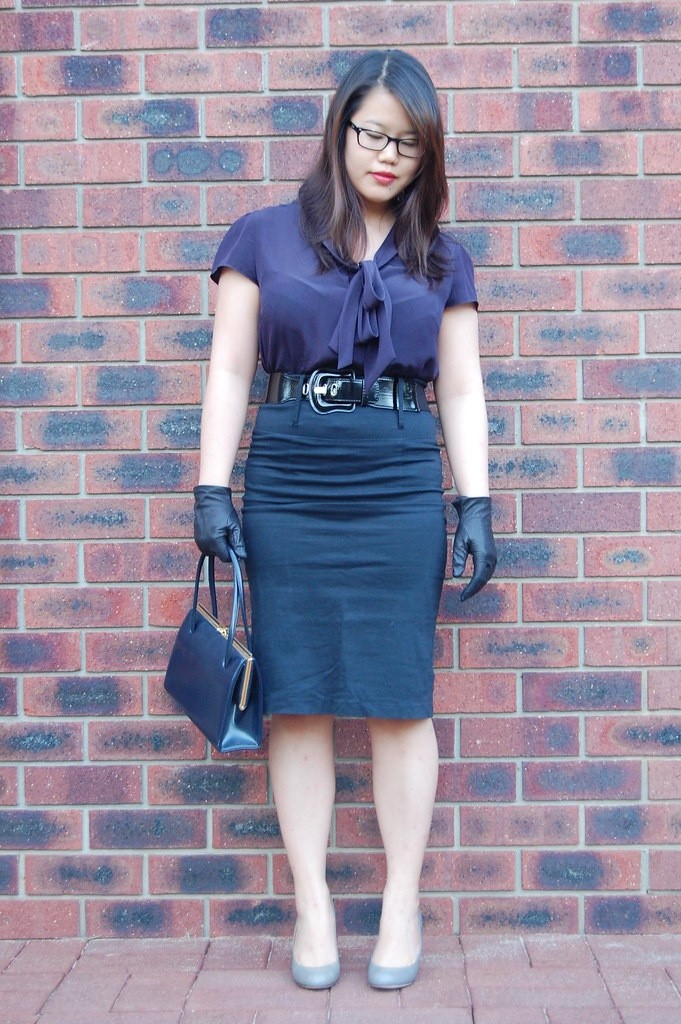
<box><xmin>290</xmin><ymin>896</ymin><xmax>342</xmax><ymax>990</ymax></box>
<box><xmin>367</xmin><ymin>909</ymin><xmax>423</xmax><ymax>989</ymax></box>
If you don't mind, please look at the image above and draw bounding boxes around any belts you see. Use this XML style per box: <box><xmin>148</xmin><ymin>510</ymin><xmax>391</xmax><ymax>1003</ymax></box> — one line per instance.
<box><xmin>265</xmin><ymin>367</ymin><xmax>430</xmax><ymax>415</ymax></box>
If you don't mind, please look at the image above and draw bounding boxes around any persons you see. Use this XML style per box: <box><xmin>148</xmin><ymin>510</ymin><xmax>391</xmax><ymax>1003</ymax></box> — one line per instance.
<box><xmin>194</xmin><ymin>49</ymin><xmax>498</xmax><ymax>990</ymax></box>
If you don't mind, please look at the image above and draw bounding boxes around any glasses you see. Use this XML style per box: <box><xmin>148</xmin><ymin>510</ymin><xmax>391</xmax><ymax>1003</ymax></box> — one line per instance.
<box><xmin>346</xmin><ymin>119</ymin><xmax>426</xmax><ymax>158</ymax></box>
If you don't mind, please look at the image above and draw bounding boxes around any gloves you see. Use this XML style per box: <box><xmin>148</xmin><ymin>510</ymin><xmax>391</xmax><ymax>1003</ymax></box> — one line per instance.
<box><xmin>192</xmin><ymin>485</ymin><xmax>247</xmax><ymax>563</ymax></box>
<box><xmin>452</xmin><ymin>496</ymin><xmax>498</xmax><ymax>602</ymax></box>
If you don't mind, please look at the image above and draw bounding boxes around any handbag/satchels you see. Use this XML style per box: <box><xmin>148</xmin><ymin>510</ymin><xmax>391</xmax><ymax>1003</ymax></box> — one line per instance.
<box><xmin>164</xmin><ymin>545</ymin><xmax>264</xmax><ymax>753</ymax></box>
